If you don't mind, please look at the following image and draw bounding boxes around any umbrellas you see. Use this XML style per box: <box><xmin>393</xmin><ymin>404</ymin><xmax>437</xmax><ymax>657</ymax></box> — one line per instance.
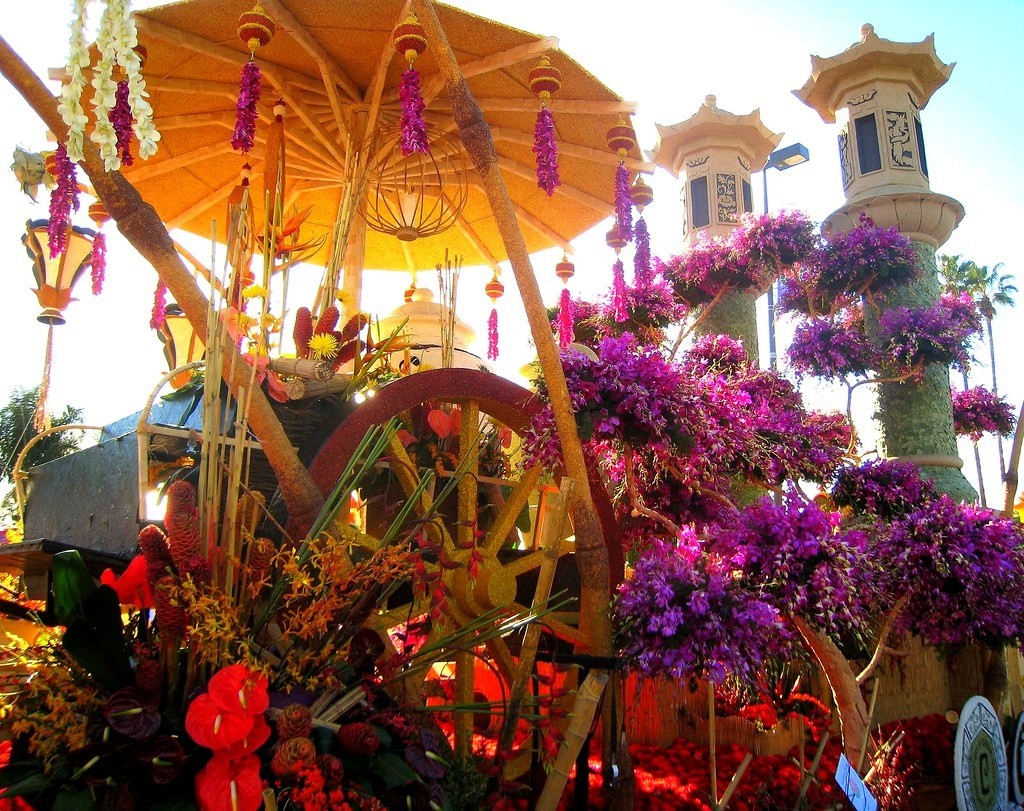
<box><xmin>47</xmin><ymin>0</ymin><xmax>652</xmax><ymax>375</ymax></box>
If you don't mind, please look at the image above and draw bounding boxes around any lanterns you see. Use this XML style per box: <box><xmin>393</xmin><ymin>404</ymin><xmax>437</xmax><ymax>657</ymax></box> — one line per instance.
<box><xmin>21</xmin><ymin>218</ymin><xmax>100</xmax><ymax>430</ymax></box>
<box><xmin>156</xmin><ymin>301</ymin><xmax>206</xmax><ymax>389</ymax></box>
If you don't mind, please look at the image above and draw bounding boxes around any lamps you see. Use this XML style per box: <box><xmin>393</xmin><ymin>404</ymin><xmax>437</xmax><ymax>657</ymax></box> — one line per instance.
<box><xmin>18</xmin><ymin>218</ymin><xmax>101</xmax><ymax>437</ymax></box>
<box><xmin>158</xmin><ymin>270</ymin><xmax>208</xmax><ymax>390</ymax></box>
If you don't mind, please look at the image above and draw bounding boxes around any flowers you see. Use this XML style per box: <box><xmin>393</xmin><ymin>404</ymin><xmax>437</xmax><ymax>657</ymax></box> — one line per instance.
<box><xmin>503</xmin><ymin>211</ymin><xmax>1024</xmax><ymax>782</ymax></box>
